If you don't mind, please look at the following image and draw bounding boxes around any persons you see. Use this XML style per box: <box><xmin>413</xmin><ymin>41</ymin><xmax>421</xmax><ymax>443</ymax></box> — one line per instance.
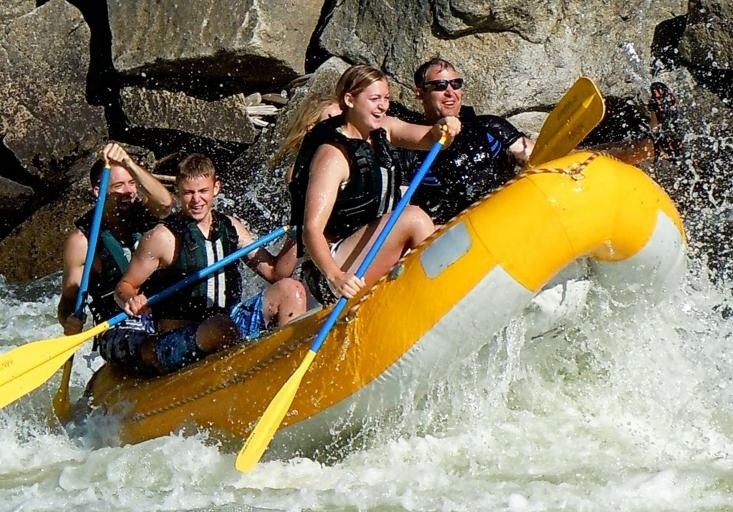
<box><xmin>273</xmin><ymin>93</ymin><xmax>346</xmax><ymax>189</ymax></box>
<box><xmin>52</xmin><ymin>141</ymin><xmax>176</xmax><ymax>378</ymax></box>
<box><xmin>393</xmin><ymin>54</ymin><xmax>538</xmax><ymax>235</ymax></box>
<box><xmin>113</xmin><ymin>152</ymin><xmax>308</xmax><ymax>371</ymax></box>
<box><xmin>291</xmin><ymin>65</ymin><xmax>462</xmax><ymax>306</ymax></box>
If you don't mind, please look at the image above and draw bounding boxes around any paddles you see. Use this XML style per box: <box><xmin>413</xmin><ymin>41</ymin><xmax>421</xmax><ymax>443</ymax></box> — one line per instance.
<box><xmin>516</xmin><ymin>77</ymin><xmax>607</xmax><ymax>174</ymax></box>
<box><xmin>47</xmin><ymin>151</ymin><xmax>111</xmax><ymax>427</ymax></box>
<box><xmin>235</xmin><ymin>124</ymin><xmax>448</xmax><ymax>471</ymax></box>
<box><xmin>0</xmin><ymin>225</ymin><xmax>289</xmax><ymax>409</ymax></box>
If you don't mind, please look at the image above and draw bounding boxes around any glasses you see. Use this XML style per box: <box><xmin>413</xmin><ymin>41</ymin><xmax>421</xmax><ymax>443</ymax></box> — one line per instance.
<box><xmin>424</xmin><ymin>78</ymin><xmax>464</xmax><ymax>91</ymax></box>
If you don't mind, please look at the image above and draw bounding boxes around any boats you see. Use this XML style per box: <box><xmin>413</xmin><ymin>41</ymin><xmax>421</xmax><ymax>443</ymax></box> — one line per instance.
<box><xmin>82</xmin><ymin>152</ymin><xmax>686</xmax><ymax>466</ymax></box>
